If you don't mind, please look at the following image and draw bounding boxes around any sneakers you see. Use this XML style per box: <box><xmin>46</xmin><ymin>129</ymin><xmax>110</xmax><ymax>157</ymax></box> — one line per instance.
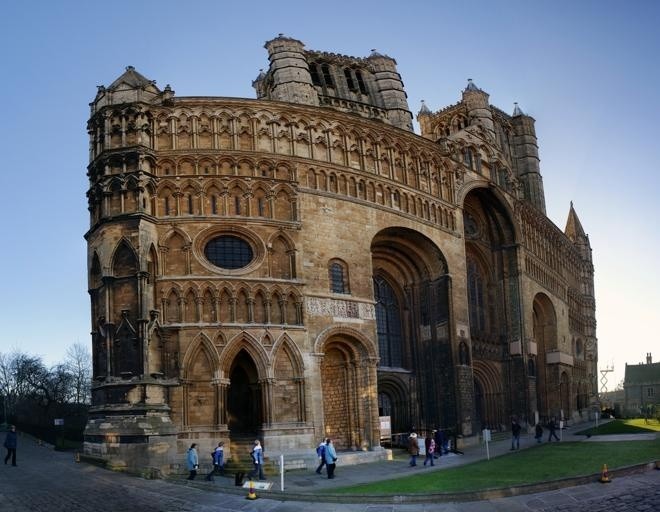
<box><xmin>248</xmin><ymin>475</ymin><xmax>251</xmax><ymax>480</ymax></box>
<box><xmin>316</xmin><ymin>470</ymin><xmax>322</xmax><ymax>474</ymax></box>
<box><xmin>207</xmin><ymin>476</ymin><xmax>211</xmax><ymax>481</ymax></box>
<box><xmin>259</xmin><ymin>477</ymin><xmax>266</xmax><ymax>480</ymax></box>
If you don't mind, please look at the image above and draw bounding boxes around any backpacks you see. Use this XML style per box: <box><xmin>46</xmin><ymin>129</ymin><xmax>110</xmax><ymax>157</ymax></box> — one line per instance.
<box><xmin>317</xmin><ymin>446</ymin><xmax>324</xmax><ymax>457</ymax></box>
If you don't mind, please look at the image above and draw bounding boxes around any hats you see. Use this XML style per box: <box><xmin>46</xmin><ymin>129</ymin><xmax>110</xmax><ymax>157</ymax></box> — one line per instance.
<box><xmin>409</xmin><ymin>432</ymin><xmax>417</xmax><ymax>438</ymax></box>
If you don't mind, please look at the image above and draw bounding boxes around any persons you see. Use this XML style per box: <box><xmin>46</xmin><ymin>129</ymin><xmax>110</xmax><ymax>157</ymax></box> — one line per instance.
<box><xmin>324</xmin><ymin>439</ymin><xmax>339</xmax><ymax>480</ymax></box>
<box><xmin>407</xmin><ymin>433</ymin><xmax>421</xmax><ymax>467</ymax></box>
<box><xmin>435</xmin><ymin>428</ymin><xmax>444</xmax><ymax>457</ymax></box>
<box><xmin>315</xmin><ymin>438</ymin><xmax>327</xmax><ymax>475</ymax></box>
<box><xmin>246</xmin><ymin>440</ymin><xmax>267</xmax><ymax>482</ymax></box>
<box><xmin>509</xmin><ymin>418</ymin><xmax>522</xmax><ymax>452</ymax></box>
<box><xmin>186</xmin><ymin>443</ymin><xmax>200</xmax><ymax>480</ymax></box>
<box><xmin>206</xmin><ymin>442</ymin><xmax>226</xmax><ymax>482</ymax></box>
<box><xmin>535</xmin><ymin>420</ymin><xmax>544</xmax><ymax>444</ymax></box>
<box><xmin>423</xmin><ymin>432</ymin><xmax>436</xmax><ymax>467</ymax></box>
<box><xmin>2</xmin><ymin>425</ymin><xmax>18</xmax><ymax>467</ymax></box>
<box><xmin>442</xmin><ymin>430</ymin><xmax>449</xmax><ymax>455</ymax></box>
<box><xmin>548</xmin><ymin>417</ymin><xmax>561</xmax><ymax>442</ymax></box>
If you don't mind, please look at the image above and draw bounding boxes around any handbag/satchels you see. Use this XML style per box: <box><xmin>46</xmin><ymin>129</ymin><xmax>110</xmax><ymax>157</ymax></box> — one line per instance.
<box><xmin>428</xmin><ymin>447</ymin><xmax>434</xmax><ymax>454</ymax></box>
<box><xmin>211</xmin><ymin>452</ymin><xmax>215</xmax><ymax>459</ymax></box>
<box><xmin>250</xmin><ymin>452</ymin><xmax>254</xmax><ymax>458</ymax></box>
